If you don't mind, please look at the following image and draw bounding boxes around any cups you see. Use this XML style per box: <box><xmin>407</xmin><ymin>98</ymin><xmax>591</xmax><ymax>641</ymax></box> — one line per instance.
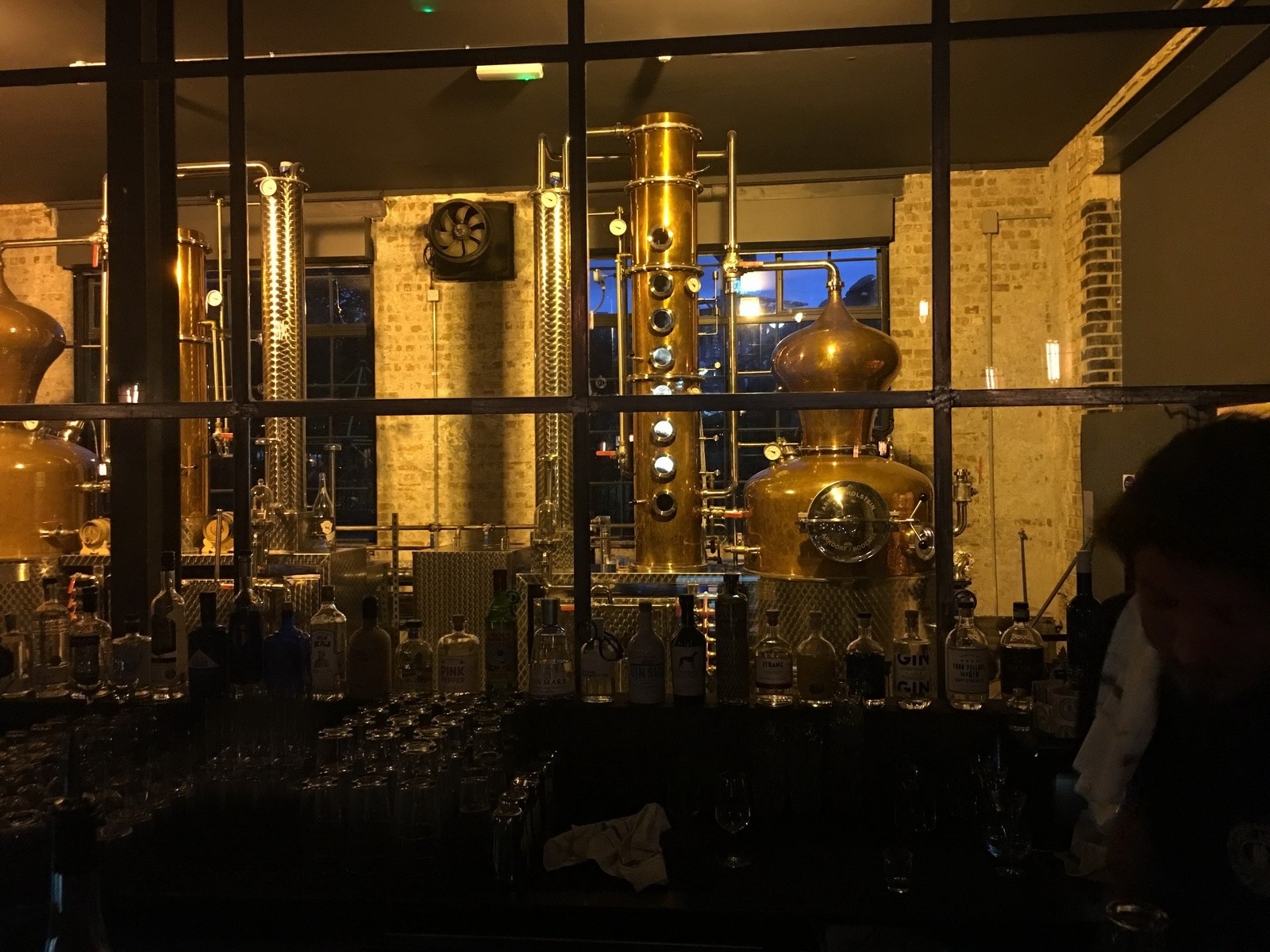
<box><xmin>0</xmin><ymin>680</ymin><xmax>1079</xmax><ymax>896</ymax></box>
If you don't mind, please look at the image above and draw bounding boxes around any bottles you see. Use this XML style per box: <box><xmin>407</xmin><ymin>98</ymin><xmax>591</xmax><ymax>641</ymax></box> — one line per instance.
<box><xmin>628</xmin><ymin>602</ymin><xmax>666</xmax><ymax>704</ymax></box>
<box><xmin>392</xmin><ymin>618</ymin><xmax>434</xmax><ymax>693</ymax></box>
<box><xmin>484</xmin><ymin>569</ymin><xmax>518</xmax><ymax>692</ymax></box>
<box><xmin>438</xmin><ymin>614</ymin><xmax>481</xmax><ymax>693</ymax></box>
<box><xmin>581</xmin><ymin>617</ymin><xmax>614</xmax><ymax>703</ymax></box>
<box><xmin>345</xmin><ymin>596</ymin><xmax>392</xmax><ymax>699</ymax></box>
<box><xmin>846</xmin><ymin>613</ymin><xmax>886</xmax><ymax>708</ymax></box>
<box><xmin>670</xmin><ymin>594</ymin><xmax>707</xmax><ymax>705</ymax></box>
<box><xmin>0</xmin><ymin>550</ymin><xmax>268</xmax><ymax>702</ymax></box>
<box><xmin>945</xmin><ymin>608</ymin><xmax>990</xmax><ymax>710</ymax></box>
<box><xmin>529</xmin><ymin>598</ymin><xmax>576</xmax><ymax>701</ymax></box>
<box><xmin>1001</xmin><ymin>602</ymin><xmax>1047</xmax><ymax>712</ymax></box>
<box><xmin>755</xmin><ymin>609</ymin><xmax>792</xmax><ymax>707</ymax></box>
<box><xmin>894</xmin><ymin>609</ymin><xmax>932</xmax><ymax>710</ymax></box>
<box><xmin>715</xmin><ymin>574</ymin><xmax>751</xmax><ymax>705</ymax></box>
<box><xmin>309</xmin><ymin>584</ymin><xmax>347</xmax><ymax>702</ymax></box>
<box><xmin>796</xmin><ymin>610</ymin><xmax>837</xmax><ymax>707</ymax></box>
<box><xmin>260</xmin><ymin>602</ymin><xmax>311</xmax><ymax>701</ymax></box>
<box><xmin>312</xmin><ymin>473</ymin><xmax>334</xmax><ymax>553</ymax></box>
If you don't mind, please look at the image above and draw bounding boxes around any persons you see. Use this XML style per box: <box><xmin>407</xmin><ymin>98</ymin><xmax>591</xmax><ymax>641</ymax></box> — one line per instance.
<box><xmin>1090</xmin><ymin>418</ymin><xmax>1270</xmax><ymax>952</ymax></box>
